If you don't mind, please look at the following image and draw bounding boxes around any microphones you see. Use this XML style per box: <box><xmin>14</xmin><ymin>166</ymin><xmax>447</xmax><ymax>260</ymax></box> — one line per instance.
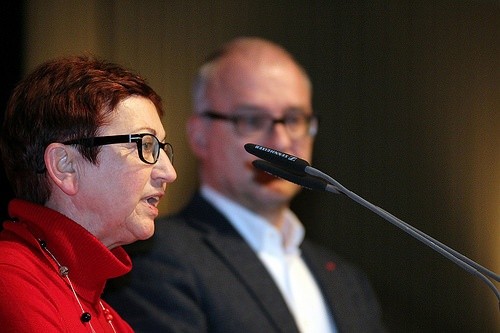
<box><xmin>242</xmin><ymin>141</ymin><xmax>500</xmax><ymax>300</ymax></box>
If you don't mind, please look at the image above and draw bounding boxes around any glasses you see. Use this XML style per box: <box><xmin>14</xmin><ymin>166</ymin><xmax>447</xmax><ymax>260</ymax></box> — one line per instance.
<box><xmin>200</xmin><ymin>110</ymin><xmax>319</xmax><ymax>138</ymax></box>
<box><xmin>60</xmin><ymin>133</ymin><xmax>174</xmax><ymax>167</ymax></box>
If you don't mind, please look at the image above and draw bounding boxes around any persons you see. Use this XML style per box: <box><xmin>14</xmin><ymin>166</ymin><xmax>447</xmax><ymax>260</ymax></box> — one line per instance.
<box><xmin>0</xmin><ymin>54</ymin><xmax>178</xmax><ymax>332</ymax></box>
<box><xmin>101</xmin><ymin>35</ymin><xmax>387</xmax><ymax>333</ymax></box>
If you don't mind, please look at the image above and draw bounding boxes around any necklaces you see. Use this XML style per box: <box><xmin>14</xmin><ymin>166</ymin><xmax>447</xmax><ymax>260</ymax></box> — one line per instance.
<box><xmin>5</xmin><ymin>216</ymin><xmax>118</xmax><ymax>333</ymax></box>
